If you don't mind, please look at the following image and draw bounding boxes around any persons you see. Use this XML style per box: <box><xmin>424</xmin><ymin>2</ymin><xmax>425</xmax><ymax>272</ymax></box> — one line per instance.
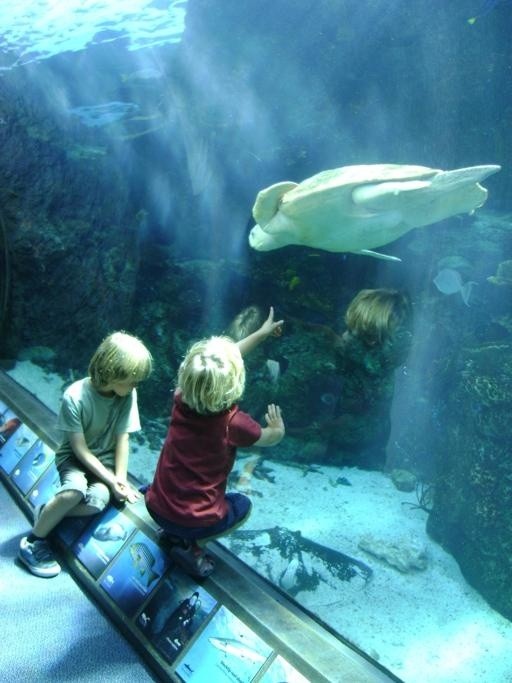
<box><xmin>17</xmin><ymin>327</ymin><xmax>156</xmax><ymax>579</ymax></box>
<box><xmin>211</xmin><ymin>302</ymin><xmax>274</xmax><ymax>494</ymax></box>
<box><xmin>160</xmin><ymin>592</ymin><xmax>201</xmax><ymax>644</ymax></box>
<box><xmin>142</xmin><ymin>301</ymin><xmax>290</xmax><ymax>580</ymax></box>
<box><xmin>328</xmin><ymin>287</ymin><xmax>412</xmax><ymax>469</ymax></box>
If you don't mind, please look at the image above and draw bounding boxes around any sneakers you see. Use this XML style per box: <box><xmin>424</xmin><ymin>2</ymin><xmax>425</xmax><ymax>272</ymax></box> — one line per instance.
<box><xmin>17</xmin><ymin>536</ymin><xmax>62</xmax><ymax>578</ymax></box>
<box><xmin>156</xmin><ymin>528</ymin><xmax>217</xmax><ymax>578</ymax></box>
